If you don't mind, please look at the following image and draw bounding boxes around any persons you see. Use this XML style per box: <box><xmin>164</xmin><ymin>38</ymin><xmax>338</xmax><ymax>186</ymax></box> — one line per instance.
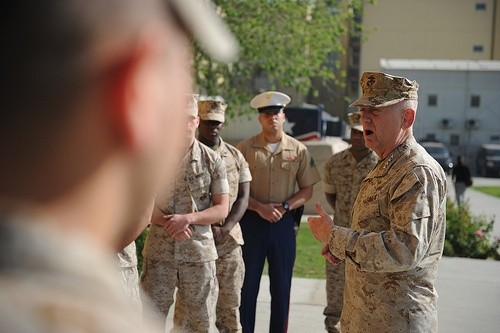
<box><xmin>139</xmin><ymin>94</ymin><xmax>230</xmax><ymax>333</ymax></box>
<box><xmin>171</xmin><ymin>95</ymin><xmax>253</xmax><ymax>333</ymax></box>
<box><xmin>307</xmin><ymin>72</ymin><xmax>447</xmax><ymax>333</ymax></box>
<box><xmin>237</xmin><ymin>91</ymin><xmax>322</xmax><ymax>333</ymax></box>
<box><xmin>323</xmin><ymin>112</ymin><xmax>379</xmax><ymax>333</ymax></box>
<box><xmin>0</xmin><ymin>0</ymin><xmax>240</xmax><ymax>333</ymax></box>
<box><xmin>452</xmin><ymin>154</ymin><xmax>472</xmax><ymax>210</ymax></box>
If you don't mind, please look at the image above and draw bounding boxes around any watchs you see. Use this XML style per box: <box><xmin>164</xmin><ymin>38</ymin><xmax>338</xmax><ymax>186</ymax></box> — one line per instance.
<box><xmin>282</xmin><ymin>201</ymin><xmax>290</xmax><ymax>212</ymax></box>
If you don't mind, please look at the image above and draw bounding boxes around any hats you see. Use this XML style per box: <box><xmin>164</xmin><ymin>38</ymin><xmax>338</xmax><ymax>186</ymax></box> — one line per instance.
<box><xmin>348</xmin><ymin>112</ymin><xmax>364</xmax><ymax>132</ymax></box>
<box><xmin>249</xmin><ymin>91</ymin><xmax>291</xmax><ymax>113</ymax></box>
<box><xmin>348</xmin><ymin>71</ymin><xmax>420</xmax><ymax>108</ymax></box>
<box><xmin>197</xmin><ymin>99</ymin><xmax>228</xmax><ymax>123</ymax></box>
<box><xmin>165</xmin><ymin>0</ymin><xmax>246</xmax><ymax>64</ymax></box>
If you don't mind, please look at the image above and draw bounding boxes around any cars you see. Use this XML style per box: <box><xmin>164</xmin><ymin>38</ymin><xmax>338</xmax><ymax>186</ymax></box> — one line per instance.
<box><xmin>477</xmin><ymin>143</ymin><xmax>500</xmax><ymax>177</ymax></box>
<box><xmin>418</xmin><ymin>142</ymin><xmax>453</xmax><ymax>172</ymax></box>
